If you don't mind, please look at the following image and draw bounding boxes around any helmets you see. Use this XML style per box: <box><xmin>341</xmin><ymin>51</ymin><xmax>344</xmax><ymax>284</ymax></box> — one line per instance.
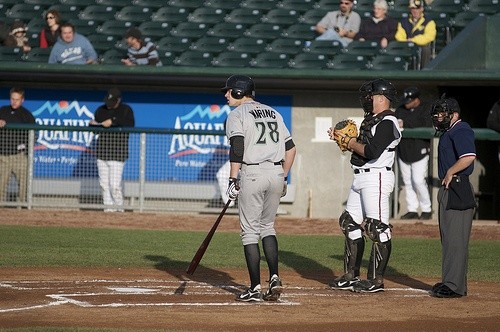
<box><xmin>432</xmin><ymin>98</ymin><xmax>460</xmax><ymax>114</ymax></box>
<box><xmin>217</xmin><ymin>74</ymin><xmax>256</xmax><ymax>99</ymax></box>
<box><xmin>361</xmin><ymin>78</ymin><xmax>393</xmax><ymax>101</ymax></box>
<box><xmin>401</xmin><ymin>87</ymin><xmax>421</xmax><ymax>104</ymax></box>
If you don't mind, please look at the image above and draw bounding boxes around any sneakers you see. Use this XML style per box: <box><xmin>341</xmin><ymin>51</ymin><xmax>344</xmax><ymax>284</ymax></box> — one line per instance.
<box><xmin>329</xmin><ymin>276</ymin><xmax>361</xmax><ymax>292</ymax></box>
<box><xmin>262</xmin><ymin>274</ymin><xmax>283</xmax><ymax>302</ymax></box>
<box><xmin>234</xmin><ymin>284</ymin><xmax>261</xmax><ymax>302</ymax></box>
<box><xmin>353</xmin><ymin>279</ymin><xmax>384</xmax><ymax>293</ymax></box>
<box><xmin>430</xmin><ymin>282</ymin><xmax>467</xmax><ymax>298</ymax></box>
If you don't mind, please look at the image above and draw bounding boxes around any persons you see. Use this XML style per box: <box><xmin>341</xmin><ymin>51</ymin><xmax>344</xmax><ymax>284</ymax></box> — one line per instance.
<box><xmin>90</xmin><ymin>91</ymin><xmax>134</xmax><ymax>212</ymax></box>
<box><xmin>394</xmin><ymin>0</ymin><xmax>437</xmax><ymax>47</ymax></box>
<box><xmin>215</xmin><ymin>160</ymin><xmax>236</xmax><ymax>208</ymax></box>
<box><xmin>39</xmin><ymin>10</ymin><xmax>64</xmax><ymax>48</ymax></box>
<box><xmin>47</xmin><ymin>22</ymin><xmax>98</xmax><ymax>65</ymax></box>
<box><xmin>220</xmin><ymin>74</ymin><xmax>296</xmax><ymax>301</ymax></box>
<box><xmin>4</xmin><ymin>20</ymin><xmax>31</xmax><ymax>58</ymax></box>
<box><xmin>327</xmin><ymin>79</ymin><xmax>401</xmax><ymax>293</ymax></box>
<box><xmin>317</xmin><ymin>0</ymin><xmax>361</xmax><ymax>49</ymax></box>
<box><xmin>393</xmin><ymin>87</ymin><xmax>433</xmax><ymax>221</ymax></box>
<box><xmin>355</xmin><ymin>0</ymin><xmax>398</xmax><ymax>49</ymax></box>
<box><xmin>430</xmin><ymin>98</ymin><xmax>479</xmax><ymax>296</ymax></box>
<box><xmin>0</xmin><ymin>87</ymin><xmax>37</xmax><ymax>208</ymax></box>
<box><xmin>120</xmin><ymin>29</ymin><xmax>162</xmax><ymax>66</ymax></box>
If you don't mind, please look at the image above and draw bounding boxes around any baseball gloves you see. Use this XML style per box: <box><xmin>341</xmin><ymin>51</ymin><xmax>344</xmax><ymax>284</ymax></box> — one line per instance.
<box><xmin>334</xmin><ymin>120</ymin><xmax>358</xmax><ymax>152</ymax></box>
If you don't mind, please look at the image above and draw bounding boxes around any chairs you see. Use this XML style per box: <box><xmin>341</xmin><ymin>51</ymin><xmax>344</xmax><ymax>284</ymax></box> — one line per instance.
<box><xmin>0</xmin><ymin>0</ymin><xmax>500</xmax><ymax>72</ymax></box>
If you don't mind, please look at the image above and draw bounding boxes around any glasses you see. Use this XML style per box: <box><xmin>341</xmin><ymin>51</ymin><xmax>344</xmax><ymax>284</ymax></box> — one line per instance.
<box><xmin>46</xmin><ymin>16</ymin><xmax>55</xmax><ymax>20</ymax></box>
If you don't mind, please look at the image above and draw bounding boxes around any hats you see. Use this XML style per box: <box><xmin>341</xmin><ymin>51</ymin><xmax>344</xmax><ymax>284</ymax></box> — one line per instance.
<box><xmin>125</xmin><ymin>28</ymin><xmax>142</xmax><ymax>39</ymax></box>
<box><xmin>408</xmin><ymin>0</ymin><xmax>424</xmax><ymax>9</ymax></box>
<box><xmin>104</xmin><ymin>87</ymin><xmax>123</xmax><ymax>105</ymax></box>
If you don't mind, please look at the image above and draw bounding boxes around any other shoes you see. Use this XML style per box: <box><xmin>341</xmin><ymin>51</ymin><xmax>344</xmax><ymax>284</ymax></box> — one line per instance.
<box><xmin>420</xmin><ymin>212</ymin><xmax>432</xmax><ymax>220</ymax></box>
<box><xmin>400</xmin><ymin>212</ymin><xmax>419</xmax><ymax>219</ymax></box>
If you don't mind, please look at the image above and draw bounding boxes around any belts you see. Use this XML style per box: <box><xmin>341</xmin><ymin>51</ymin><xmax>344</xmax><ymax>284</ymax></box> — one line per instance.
<box><xmin>354</xmin><ymin>167</ymin><xmax>391</xmax><ymax>174</ymax></box>
<box><xmin>241</xmin><ymin>160</ymin><xmax>283</xmax><ymax>165</ymax></box>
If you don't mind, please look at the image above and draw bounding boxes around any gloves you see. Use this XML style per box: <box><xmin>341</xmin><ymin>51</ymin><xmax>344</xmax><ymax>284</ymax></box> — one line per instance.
<box><xmin>226</xmin><ymin>177</ymin><xmax>240</xmax><ymax>201</ymax></box>
<box><xmin>280</xmin><ymin>176</ymin><xmax>288</xmax><ymax>198</ymax></box>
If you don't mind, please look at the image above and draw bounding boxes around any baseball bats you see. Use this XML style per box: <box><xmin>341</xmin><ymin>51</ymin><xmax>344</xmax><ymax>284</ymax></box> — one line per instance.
<box><xmin>186</xmin><ymin>186</ymin><xmax>240</xmax><ymax>275</ymax></box>
